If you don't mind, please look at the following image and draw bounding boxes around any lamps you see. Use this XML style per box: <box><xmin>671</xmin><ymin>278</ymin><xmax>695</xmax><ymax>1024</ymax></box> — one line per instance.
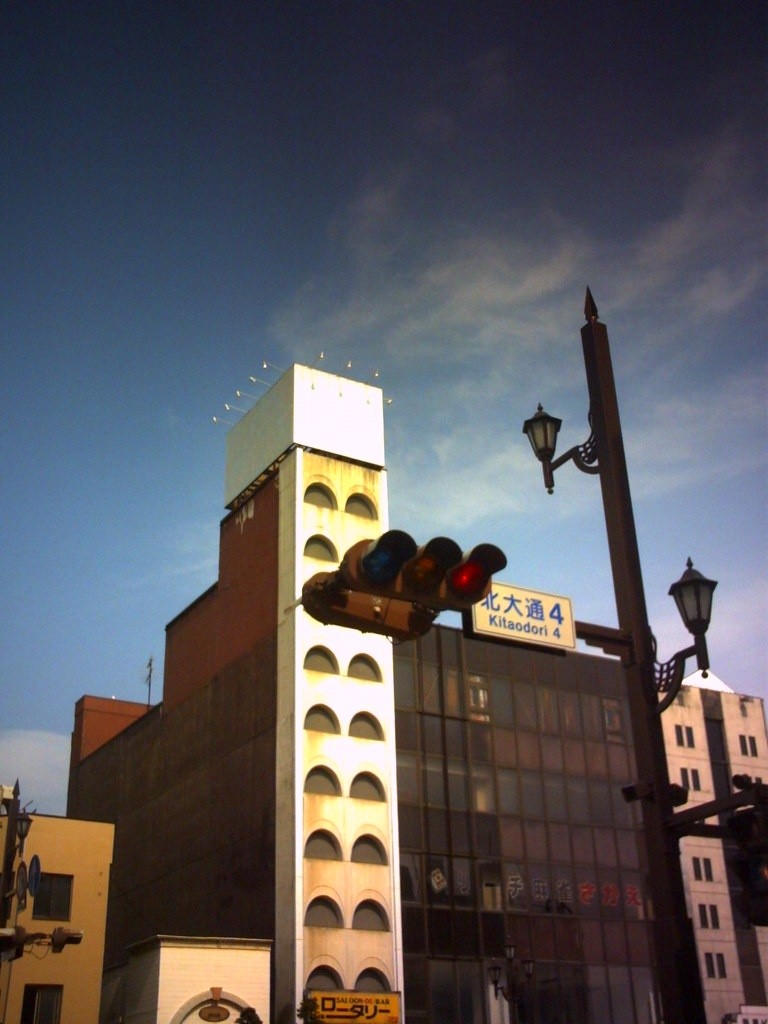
<box><xmin>248</xmin><ymin>376</ymin><xmax>271</xmax><ymax>388</ymax></box>
<box><xmin>210</xmin><ymin>415</ymin><xmax>237</xmax><ymax>426</ymax></box>
<box><xmin>311</xmin><ymin>352</ymin><xmax>325</xmax><ymax>367</ymax></box>
<box><xmin>261</xmin><ymin>361</ymin><xmax>286</xmax><ymax>373</ymax></box>
<box><xmin>234</xmin><ymin>390</ymin><xmax>259</xmax><ymax>400</ymax></box>
<box><xmin>340</xmin><ymin>360</ymin><xmax>352</xmax><ymax>376</ymax></box>
<box><xmin>364</xmin><ymin>369</ymin><xmax>381</xmax><ymax>384</ymax></box>
<box><xmin>223</xmin><ymin>401</ymin><xmax>248</xmax><ymax>414</ymax></box>
<box><xmin>383</xmin><ymin>397</ymin><xmax>393</xmax><ymax>407</ymax></box>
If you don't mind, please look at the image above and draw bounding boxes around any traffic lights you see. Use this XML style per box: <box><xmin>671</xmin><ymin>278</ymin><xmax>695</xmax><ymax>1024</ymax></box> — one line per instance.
<box><xmin>726</xmin><ymin>808</ymin><xmax>768</xmax><ymax>927</ymax></box>
<box><xmin>340</xmin><ymin>528</ymin><xmax>508</xmax><ymax>611</ymax></box>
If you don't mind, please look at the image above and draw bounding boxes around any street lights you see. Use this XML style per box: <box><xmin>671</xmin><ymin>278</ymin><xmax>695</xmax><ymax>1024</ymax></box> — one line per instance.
<box><xmin>490</xmin><ymin>933</ymin><xmax>534</xmax><ymax>1024</ymax></box>
<box><xmin>521</xmin><ymin>402</ymin><xmax>716</xmax><ymax>1024</ymax></box>
<box><xmin>0</xmin><ymin>808</ymin><xmax>33</xmax><ymax>931</ymax></box>
